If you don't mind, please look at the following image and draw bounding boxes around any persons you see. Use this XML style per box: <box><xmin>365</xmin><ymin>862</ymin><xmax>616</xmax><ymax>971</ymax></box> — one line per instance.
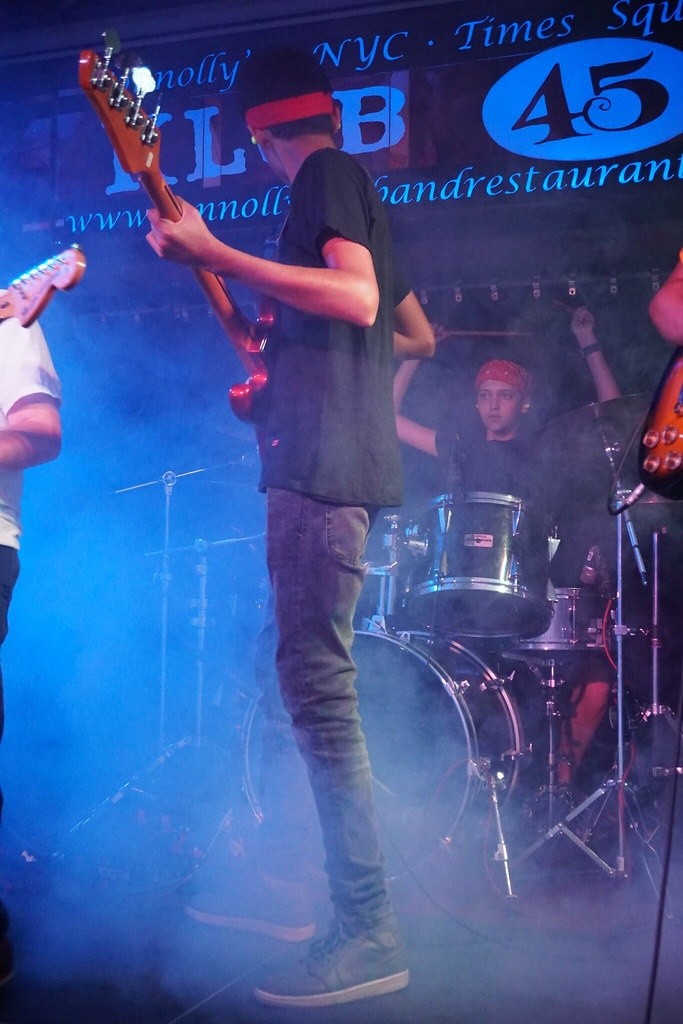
<box><xmin>392</xmin><ymin>304</ymin><xmax>639</xmax><ymax>791</ymax></box>
<box><xmin>148</xmin><ymin>47</ymin><xmax>436</xmax><ymax>1009</ymax></box>
<box><xmin>0</xmin><ymin>285</ymin><xmax>63</xmax><ymax>985</ymax></box>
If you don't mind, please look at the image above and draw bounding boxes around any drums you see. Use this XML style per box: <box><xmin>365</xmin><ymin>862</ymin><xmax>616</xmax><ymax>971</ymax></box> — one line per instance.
<box><xmin>235</xmin><ymin>627</ymin><xmax>528</xmax><ymax>888</ymax></box>
<box><xmin>390</xmin><ymin>490</ymin><xmax>557</xmax><ymax>639</ymax></box>
<box><xmin>493</xmin><ymin>590</ymin><xmax>615</xmax><ymax>653</ymax></box>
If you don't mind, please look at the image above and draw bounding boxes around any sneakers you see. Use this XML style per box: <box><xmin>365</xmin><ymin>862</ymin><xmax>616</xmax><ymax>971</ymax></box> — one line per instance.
<box><xmin>254</xmin><ymin>918</ymin><xmax>411</xmax><ymax>1009</ymax></box>
<box><xmin>184</xmin><ymin>877</ymin><xmax>317</xmax><ymax>944</ymax></box>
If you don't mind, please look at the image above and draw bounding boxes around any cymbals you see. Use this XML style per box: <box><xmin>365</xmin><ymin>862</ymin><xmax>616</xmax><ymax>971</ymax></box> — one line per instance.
<box><xmin>515</xmin><ymin>387</ymin><xmax>651</xmax><ymax>523</ymax></box>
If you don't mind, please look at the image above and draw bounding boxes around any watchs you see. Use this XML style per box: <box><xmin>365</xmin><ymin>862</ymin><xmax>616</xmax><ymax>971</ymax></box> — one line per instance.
<box><xmin>580</xmin><ymin>344</ymin><xmax>600</xmax><ymax>359</ymax></box>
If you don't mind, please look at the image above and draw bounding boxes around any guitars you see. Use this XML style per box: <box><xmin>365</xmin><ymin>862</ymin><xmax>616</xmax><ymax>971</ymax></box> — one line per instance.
<box><xmin>78</xmin><ymin>27</ymin><xmax>279</xmax><ymax>494</ymax></box>
<box><xmin>0</xmin><ymin>243</ymin><xmax>85</xmax><ymax>333</ymax></box>
<box><xmin>641</xmin><ymin>336</ymin><xmax>682</xmax><ymax>504</ymax></box>
